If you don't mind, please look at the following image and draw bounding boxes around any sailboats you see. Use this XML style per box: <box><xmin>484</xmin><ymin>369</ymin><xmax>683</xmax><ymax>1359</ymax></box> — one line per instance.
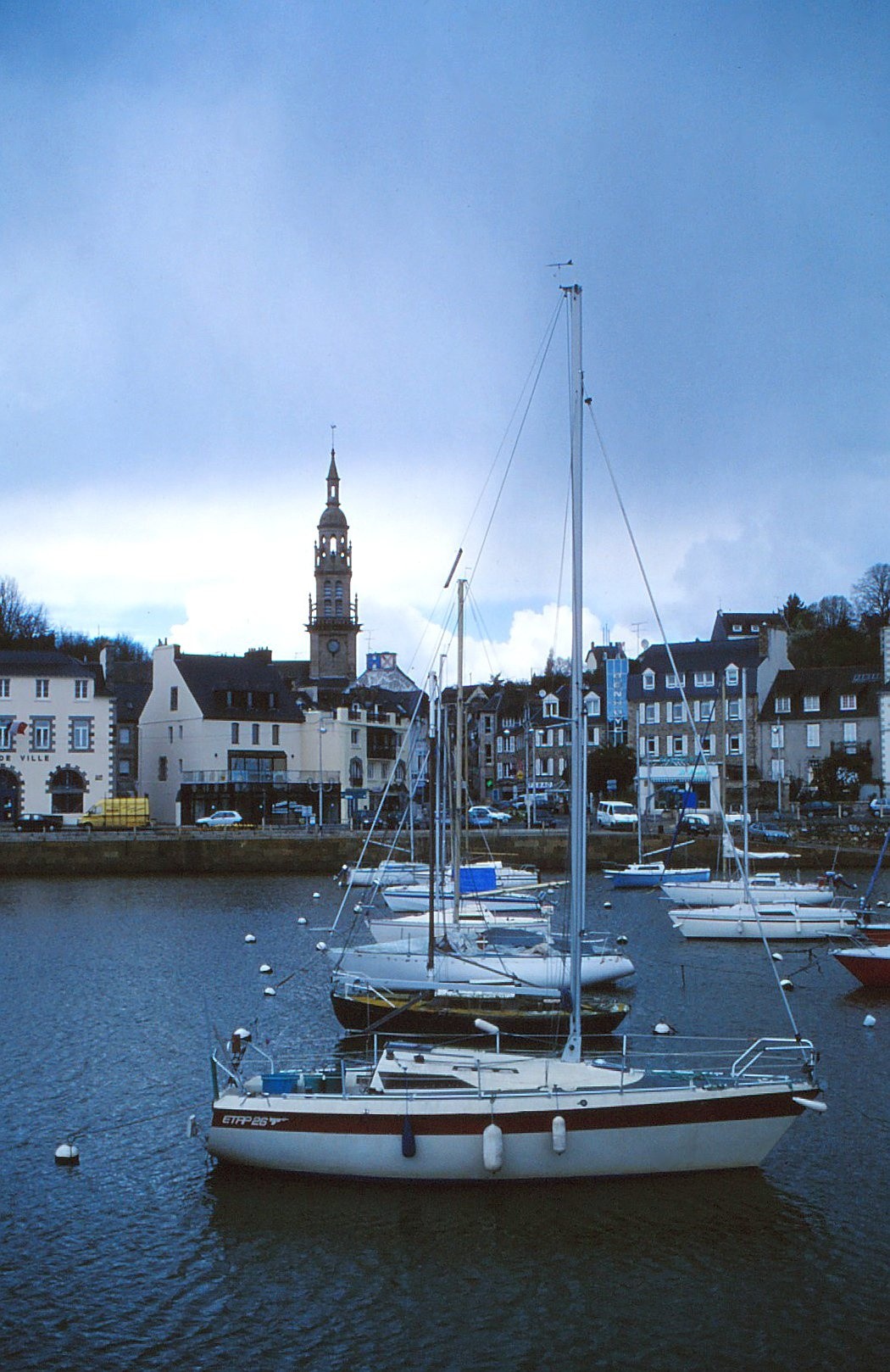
<box><xmin>335</xmin><ymin>576</ymin><xmax>630</xmax><ymax>997</ymax></box>
<box><xmin>202</xmin><ymin>284</ymin><xmax>828</xmax><ymax>1182</ymax></box>
<box><xmin>348</xmin><ymin>859</ymin><xmax>559</xmax><ymax>946</ymax></box>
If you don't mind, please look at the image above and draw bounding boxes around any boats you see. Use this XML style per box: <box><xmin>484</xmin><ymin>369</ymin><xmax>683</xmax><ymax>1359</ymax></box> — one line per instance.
<box><xmin>668</xmin><ymin>902</ymin><xmax>864</xmax><ymax>945</ymax></box>
<box><xmin>656</xmin><ymin>872</ymin><xmax>838</xmax><ymax>904</ymax></box>
<box><xmin>601</xmin><ymin>866</ymin><xmax>712</xmax><ymax>891</ymax></box>
<box><xmin>830</xmin><ymin>922</ymin><xmax>890</xmax><ymax>986</ymax></box>
<box><xmin>333</xmin><ymin>992</ymin><xmax>630</xmax><ymax>1040</ymax></box>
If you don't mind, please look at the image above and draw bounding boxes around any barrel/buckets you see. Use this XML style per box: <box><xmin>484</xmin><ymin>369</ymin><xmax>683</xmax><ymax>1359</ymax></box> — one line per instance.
<box><xmin>261</xmin><ymin>1073</ymin><xmax>299</xmax><ymax>1094</ymax></box>
<box><xmin>303</xmin><ymin>1073</ymin><xmax>342</xmax><ymax>1094</ymax></box>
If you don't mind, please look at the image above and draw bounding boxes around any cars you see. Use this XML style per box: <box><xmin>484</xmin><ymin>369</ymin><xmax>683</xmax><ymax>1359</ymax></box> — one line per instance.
<box><xmin>196</xmin><ymin>809</ymin><xmax>242</xmax><ymax>829</ymax></box>
<box><xmin>747</xmin><ymin>821</ymin><xmax>791</xmax><ymax>842</ymax></box>
<box><xmin>467</xmin><ymin>806</ymin><xmax>511</xmax><ymax>825</ymax></box>
<box><xmin>798</xmin><ymin>802</ymin><xmax>853</xmax><ymax>819</ymax></box>
<box><xmin>509</xmin><ymin>794</ymin><xmax>548</xmax><ymax>810</ymax></box>
<box><xmin>14</xmin><ymin>814</ymin><xmax>62</xmax><ymax>831</ymax></box>
<box><xmin>351</xmin><ymin>809</ymin><xmax>382</xmax><ymax>830</ymax></box>
<box><xmin>870</xmin><ymin>798</ymin><xmax>889</xmax><ymax>817</ymax></box>
<box><xmin>676</xmin><ymin>816</ymin><xmax>711</xmax><ymax>838</ymax></box>
<box><xmin>74</xmin><ymin>798</ymin><xmax>150</xmax><ymax>829</ymax></box>
<box><xmin>598</xmin><ymin>800</ymin><xmax>638</xmax><ymax>831</ymax></box>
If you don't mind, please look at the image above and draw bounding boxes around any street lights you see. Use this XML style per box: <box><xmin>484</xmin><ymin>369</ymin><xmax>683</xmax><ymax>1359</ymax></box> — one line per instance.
<box><xmin>317</xmin><ymin>716</ymin><xmax>325</xmax><ymax>838</ymax></box>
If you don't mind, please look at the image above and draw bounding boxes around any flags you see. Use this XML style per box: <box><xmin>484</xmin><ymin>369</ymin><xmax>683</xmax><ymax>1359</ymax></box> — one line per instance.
<box><xmin>5</xmin><ymin>721</ymin><xmax>28</xmax><ymax>735</ymax></box>
